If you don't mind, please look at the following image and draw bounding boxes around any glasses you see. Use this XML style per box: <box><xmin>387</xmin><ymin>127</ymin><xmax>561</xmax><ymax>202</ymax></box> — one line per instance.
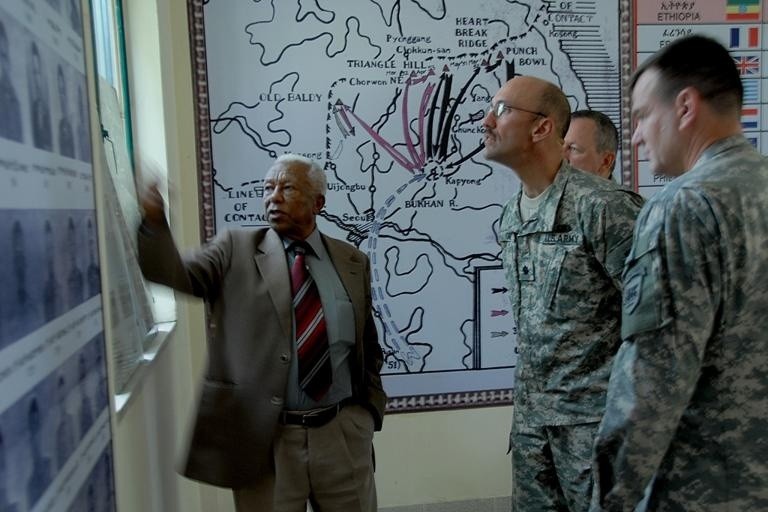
<box><xmin>484</xmin><ymin>100</ymin><xmax>547</xmax><ymax>117</ymax></box>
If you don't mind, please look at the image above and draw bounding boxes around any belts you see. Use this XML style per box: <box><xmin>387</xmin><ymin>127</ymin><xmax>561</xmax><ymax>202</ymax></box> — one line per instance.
<box><xmin>281</xmin><ymin>404</ymin><xmax>344</xmax><ymax>429</ymax></box>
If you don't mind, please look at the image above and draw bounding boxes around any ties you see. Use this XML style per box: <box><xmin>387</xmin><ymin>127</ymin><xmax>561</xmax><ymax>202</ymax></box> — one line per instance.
<box><xmin>290</xmin><ymin>244</ymin><xmax>333</xmax><ymax>404</ymax></box>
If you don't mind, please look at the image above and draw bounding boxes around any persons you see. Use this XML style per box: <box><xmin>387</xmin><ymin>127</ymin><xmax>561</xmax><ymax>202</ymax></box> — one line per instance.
<box><xmin>137</xmin><ymin>153</ymin><xmax>387</xmax><ymax>512</ymax></box>
<box><xmin>562</xmin><ymin>110</ymin><xmax>619</xmax><ymax>184</ymax></box>
<box><xmin>588</xmin><ymin>34</ymin><xmax>768</xmax><ymax>512</ymax></box>
<box><xmin>0</xmin><ymin>0</ymin><xmax>114</xmax><ymax>512</ymax></box>
<box><xmin>482</xmin><ymin>76</ymin><xmax>649</xmax><ymax>511</ymax></box>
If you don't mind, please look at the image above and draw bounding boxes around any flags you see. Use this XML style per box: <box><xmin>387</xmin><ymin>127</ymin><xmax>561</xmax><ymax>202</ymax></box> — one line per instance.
<box><xmin>724</xmin><ymin>0</ymin><xmax>761</xmax><ymax>153</ymax></box>
